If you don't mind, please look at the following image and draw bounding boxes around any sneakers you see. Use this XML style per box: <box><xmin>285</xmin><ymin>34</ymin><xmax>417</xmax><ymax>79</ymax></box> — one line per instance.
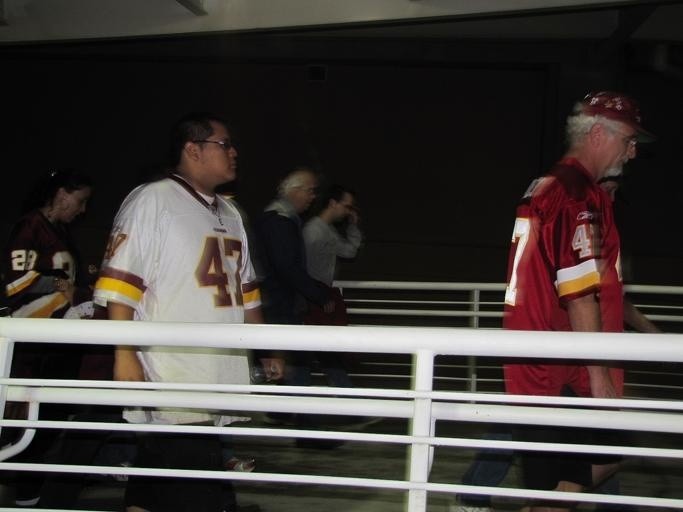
<box><xmin>226</xmin><ymin>456</ymin><xmax>256</xmax><ymax>483</ymax></box>
<box><xmin>328</xmin><ymin>414</ymin><xmax>385</xmax><ymax>431</ymax></box>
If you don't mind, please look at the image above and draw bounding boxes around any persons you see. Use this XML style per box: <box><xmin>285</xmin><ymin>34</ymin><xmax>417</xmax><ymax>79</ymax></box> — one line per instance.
<box><xmin>0</xmin><ymin>165</ymin><xmax>367</xmax><ymax>512</ymax></box>
<box><xmin>452</xmin><ymin>176</ymin><xmax>663</xmax><ymax>511</ymax></box>
<box><xmin>93</xmin><ymin>114</ymin><xmax>287</xmax><ymax>511</ymax></box>
<box><xmin>501</xmin><ymin>90</ymin><xmax>647</xmax><ymax>512</ymax></box>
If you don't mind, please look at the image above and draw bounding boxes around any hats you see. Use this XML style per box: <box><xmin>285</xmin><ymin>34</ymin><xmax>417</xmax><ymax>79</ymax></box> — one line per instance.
<box><xmin>577</xmin><ymin>86</ymin><xmax>656</xmax><ymax>145</ymax></box>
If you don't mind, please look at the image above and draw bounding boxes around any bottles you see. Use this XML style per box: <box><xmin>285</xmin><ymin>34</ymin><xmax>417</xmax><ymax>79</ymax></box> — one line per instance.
<box><xmin>249</xmin><ymin>364</ymin><xmax>277</xmax><ymax>385</ymax></box>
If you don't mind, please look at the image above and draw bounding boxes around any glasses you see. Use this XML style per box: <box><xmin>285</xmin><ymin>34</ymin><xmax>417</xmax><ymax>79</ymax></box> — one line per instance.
<box><xmin>191</xmin><ymin>137</ymin><xmax>238</xmax><ymax>149</ymax></box>
